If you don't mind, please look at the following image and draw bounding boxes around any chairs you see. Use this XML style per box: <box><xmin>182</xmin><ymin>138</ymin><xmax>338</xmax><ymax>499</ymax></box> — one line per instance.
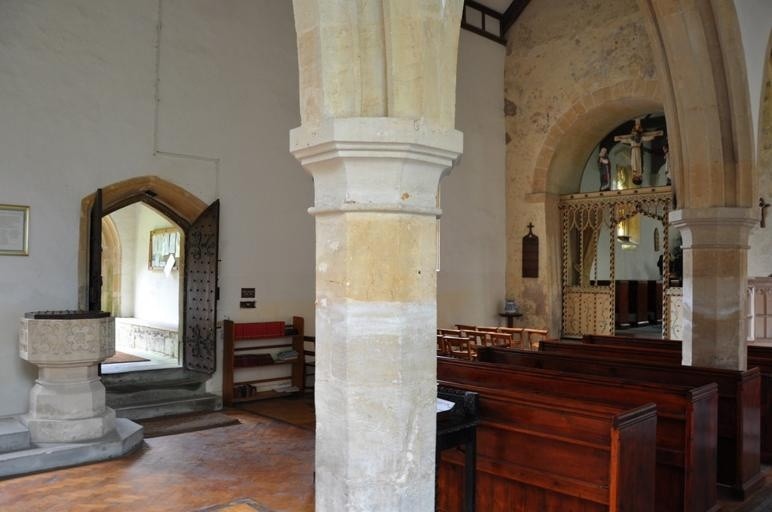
<box><xmin>436</xmin><ymin>320</ymin><xmax>547</xmax><ymax>358</ymax></box>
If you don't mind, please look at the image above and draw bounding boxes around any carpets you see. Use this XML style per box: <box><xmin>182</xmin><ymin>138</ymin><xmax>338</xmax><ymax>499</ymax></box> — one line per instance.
<box><xmin>239</xmin><ymin>398</ymin><xmax>316</xmax><ymax>432</ymax></box>
<box><xmin>101</xmin><ymin>343</ymin><xmax>150</xmax><ymax>364</ymax></box>
<box><xmin>193</xmin><ymin>495</ymin><xmax>275</xmax><ymax>512</ymax></box>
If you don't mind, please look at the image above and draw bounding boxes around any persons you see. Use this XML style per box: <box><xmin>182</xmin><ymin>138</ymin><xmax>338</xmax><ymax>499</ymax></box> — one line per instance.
<box><xmin>661</xmin><ymin>144</ymin><xmax>672</xmax><ymax>187</ymax></box>
<box><xmin>595</xmin><ymin>147</ymin><xmax>611</xmax><ymax>193</ymax></box>
<box><xmin>614</xmin><ymin>124</ymin><xmax>660</xmax><ymax>176</ymax></box>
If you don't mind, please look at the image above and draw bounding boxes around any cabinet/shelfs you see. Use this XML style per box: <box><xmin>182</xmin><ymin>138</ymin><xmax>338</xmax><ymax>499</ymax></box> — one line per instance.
<box><xmin>222</xmin><ymin>316</ymin><xmax>307</xmax><ymax>407</ymax></box>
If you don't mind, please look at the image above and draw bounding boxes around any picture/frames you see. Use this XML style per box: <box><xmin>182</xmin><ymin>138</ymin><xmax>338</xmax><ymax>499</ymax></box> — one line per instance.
<box><xmin>1</xmin><ymin>202</ymin><xmax>30</xmax><ymax>257</ymax></box>
